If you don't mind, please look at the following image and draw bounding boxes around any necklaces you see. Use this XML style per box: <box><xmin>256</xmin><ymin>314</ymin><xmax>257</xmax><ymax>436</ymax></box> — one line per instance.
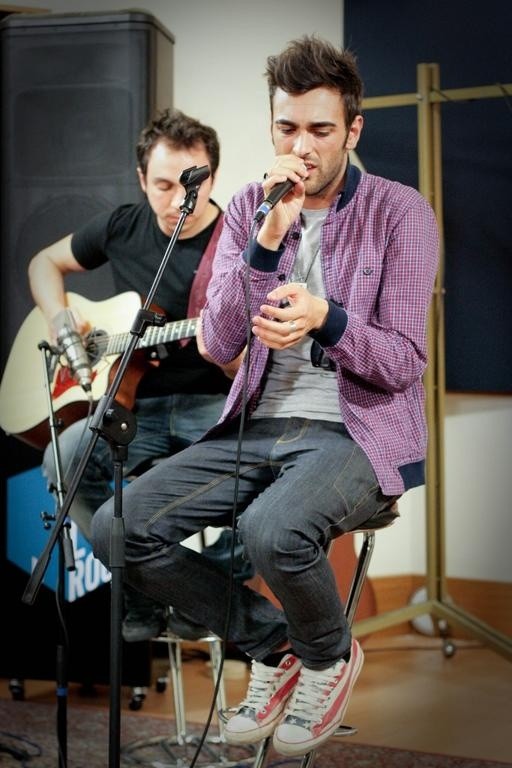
<box><xmin>295</xmin><ymin>245</ymin><xmax>321</xmax><ymax>283</ymax></box>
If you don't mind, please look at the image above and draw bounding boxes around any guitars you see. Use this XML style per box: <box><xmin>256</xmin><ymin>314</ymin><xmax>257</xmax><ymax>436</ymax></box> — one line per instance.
<box><xmin>0</xmin><ymin>292</ymin><xmax>200</xmax><ymax>451</ymax></box>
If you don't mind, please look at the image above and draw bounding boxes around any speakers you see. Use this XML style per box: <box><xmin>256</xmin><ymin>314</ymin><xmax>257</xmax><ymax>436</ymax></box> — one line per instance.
<box><xmin>0</xmin><ymin>7</ymin><xmax>176</xmax><ymax>341</ymax></box>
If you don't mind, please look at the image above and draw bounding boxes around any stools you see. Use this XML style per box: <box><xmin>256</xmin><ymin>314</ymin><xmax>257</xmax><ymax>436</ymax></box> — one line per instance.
<box><xmin>120</xmin><ymin>503</ymin><xmax>401</xmax><ymax>767</ymax></box>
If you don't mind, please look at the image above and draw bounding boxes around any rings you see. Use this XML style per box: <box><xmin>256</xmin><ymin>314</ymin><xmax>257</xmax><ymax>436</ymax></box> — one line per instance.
<box><xmin>288</xmin><ymin>321</ymin><xmax>297</xmax><ymax>333</ymax></box>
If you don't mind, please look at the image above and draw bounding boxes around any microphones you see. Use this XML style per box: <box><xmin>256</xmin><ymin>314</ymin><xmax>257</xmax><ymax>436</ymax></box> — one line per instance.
<box><xmin>51</xmin><ymin>309</ymin><xmax>93</xmax><ymax>391</ymax></box>
<box><xmin>254</xmin><ymin>176</ymin><xmax>294</xmax><ymax>223</ymax></box>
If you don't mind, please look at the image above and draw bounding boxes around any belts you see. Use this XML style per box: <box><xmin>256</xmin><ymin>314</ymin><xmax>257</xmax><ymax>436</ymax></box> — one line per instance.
<box><xmin>122</xmin><ymin>606</ymin><xmax>212</xmax><ymax>647</ymax></box>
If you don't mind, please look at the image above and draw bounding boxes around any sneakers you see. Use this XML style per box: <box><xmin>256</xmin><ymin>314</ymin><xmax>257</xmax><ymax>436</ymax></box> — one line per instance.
<box><xmin>223</xmin><ymin>654</ymin><xmax>302</xmax><ymax>746</ymax></box>
<box><xmin>273</xmin><ymin>639</ymin><xmax>365</xmax><ymax>756</ymax></box>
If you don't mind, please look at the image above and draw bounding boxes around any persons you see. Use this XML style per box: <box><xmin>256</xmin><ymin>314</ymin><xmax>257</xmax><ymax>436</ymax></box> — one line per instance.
<box><xmin>28</xmin><ymin>110</ymin><xmax>248</xmax><ymax>642</ymax></box>
<box><xmin>92</xmin><ymin>31</ymin><xmax>440</xmax><ymax>757</ymax></box>
